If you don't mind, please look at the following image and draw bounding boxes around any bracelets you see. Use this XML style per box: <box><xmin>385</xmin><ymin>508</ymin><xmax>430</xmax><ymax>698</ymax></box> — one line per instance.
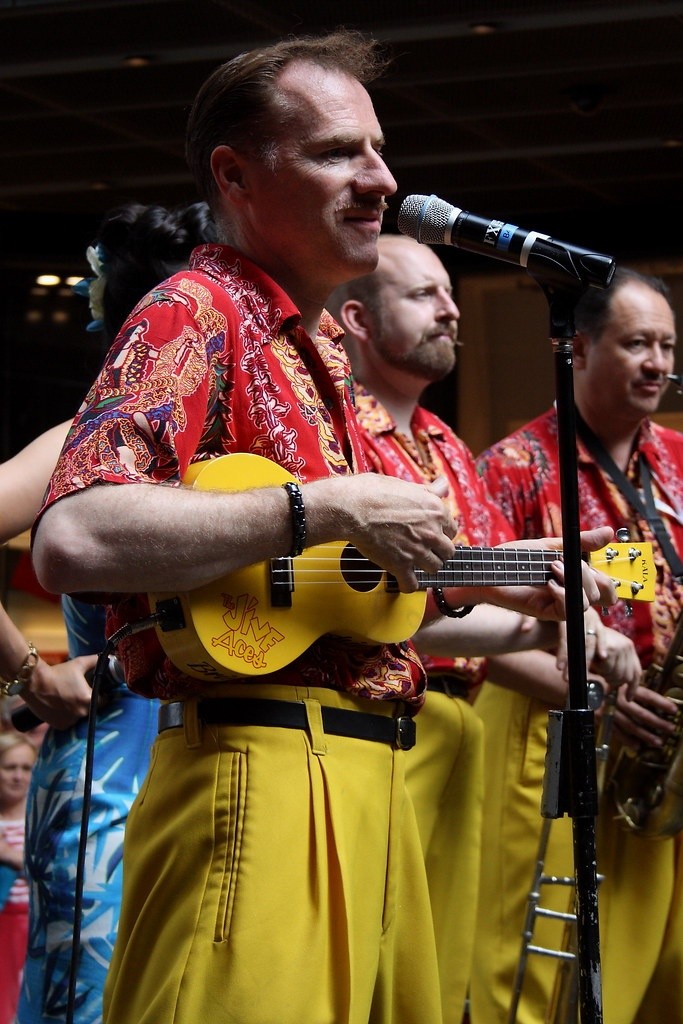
<box><xmin>432</xmin><ymin>545</ymin><xmax>477</xmax><ymax>619</ymax></box>
<box><xmin>281</xmin><ymin>481</ymin><xmax>307</xmax><ymax>558</ymax></box>
<box><xmin>0</xmin><ymin>642</ymin><xmax>39</xmax><ymax>696</ymax></box>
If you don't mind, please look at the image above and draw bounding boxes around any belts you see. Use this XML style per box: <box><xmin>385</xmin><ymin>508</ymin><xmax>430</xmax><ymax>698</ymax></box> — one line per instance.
<box><xmin>157</xmin><ymin>693</ymin><xmax>417</xmax><ymax>751</ymax></box>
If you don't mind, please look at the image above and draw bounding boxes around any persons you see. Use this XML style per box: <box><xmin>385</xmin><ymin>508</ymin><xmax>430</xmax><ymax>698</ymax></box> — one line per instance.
<box><xmin>474</xmin><ymin>267</ymin><xmax>683</xmax><ymax>1024</ymax></box>
<box><xmin>0</xmin><ymin>199</ymin><xmax>255</xmax><ymax>1024</ymax></box>
<box><xmin>29</xmin><ymin>32</ymin><xmax>619</xmax><ymax>1024</ymax></box>
<box><xmin>1</xmin><ymin>733</ymin><xmax>39</xmax><ymax>916</ymax></box>
<box><xmin>321</xmin><ymin>234</ymin><xmax>639</xmax><ymax>1024</ymax></box>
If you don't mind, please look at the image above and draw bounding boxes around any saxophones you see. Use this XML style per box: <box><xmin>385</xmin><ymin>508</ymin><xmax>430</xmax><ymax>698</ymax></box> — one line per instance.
<box><xmin>596</xmin><ymin>616</ymin><xmax>683</xmax><ymax>837</ymax></box>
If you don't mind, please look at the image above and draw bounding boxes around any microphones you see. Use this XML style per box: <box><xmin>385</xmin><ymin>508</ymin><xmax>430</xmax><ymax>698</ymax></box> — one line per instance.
<box><xmin>8</xmin><ymin>653</ymin><xmax>125</xmax><ymax>735</ymax></box>
<box><xmin>394</xmin><ymin>191</ymin><xmax>618</xmax><ymax>290</ymax></box>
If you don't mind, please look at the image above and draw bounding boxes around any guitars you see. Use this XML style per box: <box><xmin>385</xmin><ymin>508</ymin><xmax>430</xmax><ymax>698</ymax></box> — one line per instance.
<box><xmin>147</xmin><ymin>452</ymin><xmax>660</xmax><ymax>685</ymax></box>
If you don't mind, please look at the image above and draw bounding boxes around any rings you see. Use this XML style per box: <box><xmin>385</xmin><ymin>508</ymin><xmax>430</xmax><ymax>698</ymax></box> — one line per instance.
<box><xmin>586</xmin><ymin>629</ymin><xmax>596</xmax><ymax>636</ymax></box>
